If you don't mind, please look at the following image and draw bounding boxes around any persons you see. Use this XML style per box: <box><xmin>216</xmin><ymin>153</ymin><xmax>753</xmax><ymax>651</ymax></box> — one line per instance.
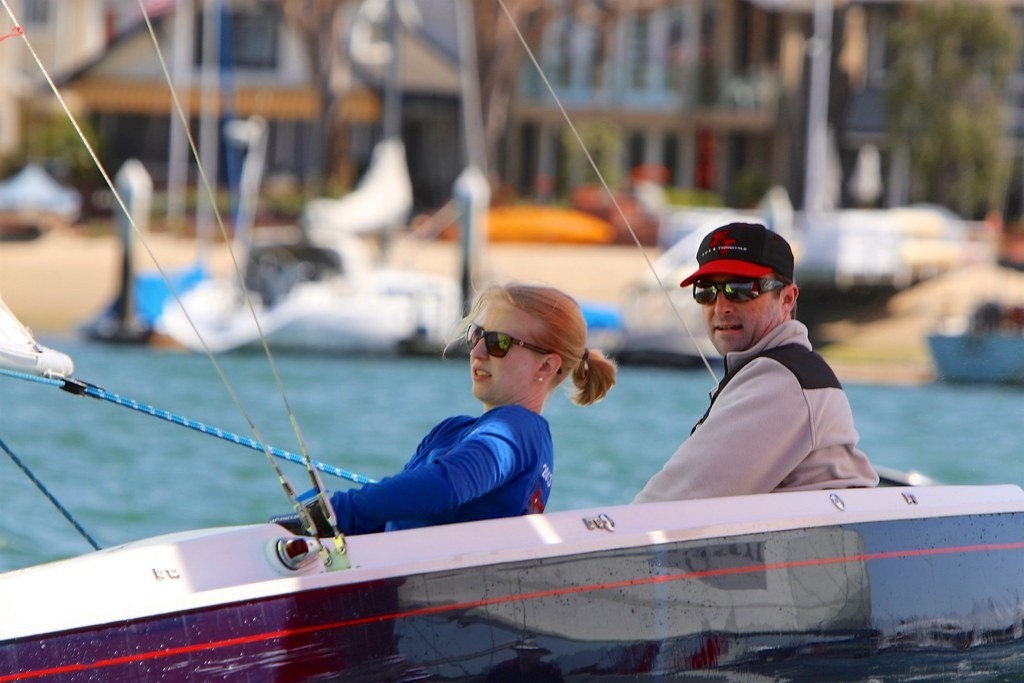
<box><xmin>623</xmin><ymin>222</ymin><xmax>879</xmax><ymax>506</ymax></box>
<box><xmin>269</xmin><ymin>282</ymin><xmax>618</xmax><ymax>538</ymax></box>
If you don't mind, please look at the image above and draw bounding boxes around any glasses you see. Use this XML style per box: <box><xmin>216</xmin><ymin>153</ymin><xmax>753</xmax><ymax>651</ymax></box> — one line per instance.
<box><xmin>466</xmin><ymin>323</ymin><xmax>562</xmax><ymax>374</ymax></box>
<box><xmin>693</xmin><ymin>278</ymin><xmax>788</xmax><ymax>304</ymax></box>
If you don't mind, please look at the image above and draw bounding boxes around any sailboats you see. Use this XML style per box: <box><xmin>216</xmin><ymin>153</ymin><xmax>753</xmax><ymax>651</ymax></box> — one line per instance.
<box><xmin>0</xmin><ymin>0</ymin><xmax>1024</xmax><ymax>683</ymax></box>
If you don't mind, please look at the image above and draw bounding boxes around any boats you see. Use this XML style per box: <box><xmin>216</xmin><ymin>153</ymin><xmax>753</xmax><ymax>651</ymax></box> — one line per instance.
<box><xmin>927</xmin><ymin>332</ymin><xmax>1024</xmax><ymax>380</ymax></box>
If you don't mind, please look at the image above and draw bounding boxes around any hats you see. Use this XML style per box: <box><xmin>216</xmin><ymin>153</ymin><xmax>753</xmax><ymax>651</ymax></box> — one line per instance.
<box><xmin>680</xmin><ymin>222</ymin><xmax>794</xmax><ymax>288</ymax></box>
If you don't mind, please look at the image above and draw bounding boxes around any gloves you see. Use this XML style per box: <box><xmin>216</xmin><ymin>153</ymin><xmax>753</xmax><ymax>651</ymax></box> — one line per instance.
<box><xmin>269</xmin><ymin>502</ymin><xmax>322</xmax><ymax>537</ymax></box>
